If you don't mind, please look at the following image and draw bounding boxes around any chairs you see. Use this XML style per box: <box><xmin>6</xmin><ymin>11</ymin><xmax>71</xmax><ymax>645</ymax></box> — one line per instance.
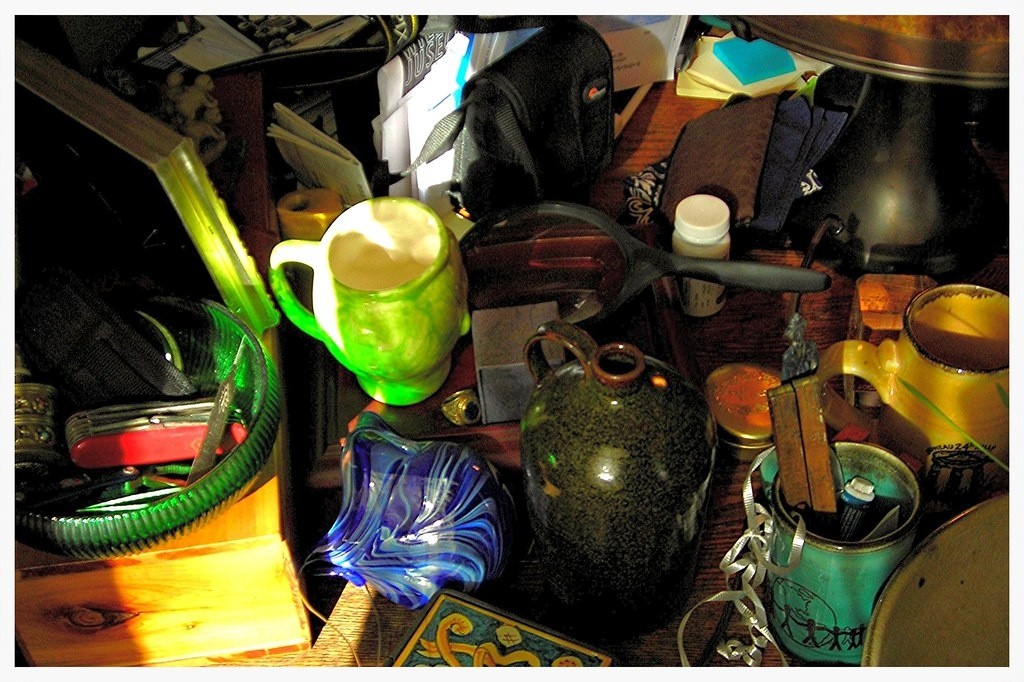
<box><xmin>208</xmin><ymin>45</ymin><xmax>705</xmax><ymax>472</ymax></box>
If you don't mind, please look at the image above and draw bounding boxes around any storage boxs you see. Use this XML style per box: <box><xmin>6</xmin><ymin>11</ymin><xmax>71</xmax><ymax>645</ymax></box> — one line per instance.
<box><xmin>15</xmin><ymin>325</ymin><xmax>311</xmax><ymax>667</ymax></box>
<box><xmin>470</xmin><ymin>302</ymin><xmax>567</xmax><ymax>423</ymax></box>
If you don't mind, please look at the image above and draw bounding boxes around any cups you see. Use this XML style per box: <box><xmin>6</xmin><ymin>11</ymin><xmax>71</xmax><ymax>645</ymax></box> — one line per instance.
<box><xmin>268</xmin><ymin>196</ymin><xmax>468</xmax><ymax>406</ymax></box>
<box><xmin>758</xmin><ymin>440</ymin><xmax>922</xmax><ymax>666</ymax></box>
<box><xmin>815</xmin><ymin>283</ymin><xmax>1009</xmax><ymax>529</ymax></box>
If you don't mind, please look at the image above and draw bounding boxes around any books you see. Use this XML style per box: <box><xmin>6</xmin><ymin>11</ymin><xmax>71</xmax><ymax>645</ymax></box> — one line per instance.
<box><xmin>674</xmin><ymin>30</ymin><xmax>797</xmax><ymax>99</ymax></box>
<box><xmin>214</xmin><ymin>15</ymin><xmax>345</xmax><ymax>51</ymax></box>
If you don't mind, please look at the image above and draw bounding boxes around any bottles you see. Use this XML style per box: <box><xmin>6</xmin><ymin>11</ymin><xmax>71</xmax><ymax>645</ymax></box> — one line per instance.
<box><xmin>672</xmin><ymin>194</ymin><xmax>731</xmax><ymax>316</ymax></box>
<box><xmin>519</xmin><ymin>317</ymin><xmax>715</xmax><ymax>623</ymax></box>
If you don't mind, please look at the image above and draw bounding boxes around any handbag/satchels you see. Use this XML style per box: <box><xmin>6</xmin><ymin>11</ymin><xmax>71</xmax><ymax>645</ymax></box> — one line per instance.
<box><xmin>451</xmin><ymin>16</ymin><xmax>616</xmax><ymax>222</ymax></box>
